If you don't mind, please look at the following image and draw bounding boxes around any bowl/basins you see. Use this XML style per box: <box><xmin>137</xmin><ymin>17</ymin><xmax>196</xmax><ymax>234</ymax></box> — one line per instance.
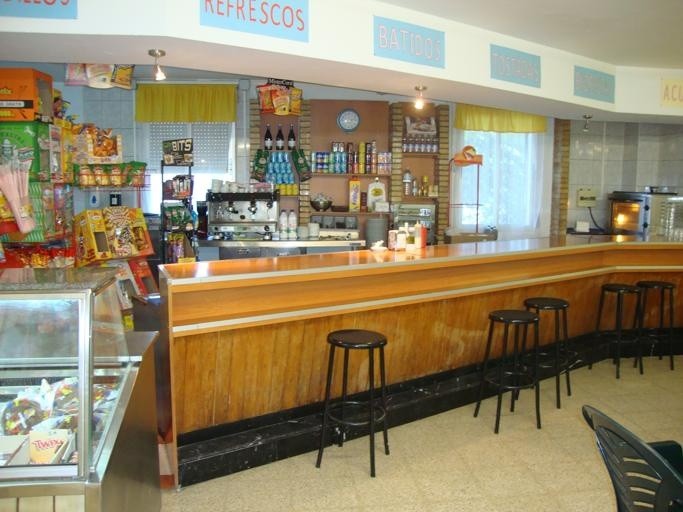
<box><xmin>310</xmin><ymin>201</ymin><xmax>332</xmax><ymax>212</ymax></box>
<box><xmin>650</xmin><ymin>185</ymin><xmax>676</xmax><ymax>193</ymax></box>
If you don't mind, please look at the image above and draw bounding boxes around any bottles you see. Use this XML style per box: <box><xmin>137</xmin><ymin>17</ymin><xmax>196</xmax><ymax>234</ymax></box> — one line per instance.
<box><xmin>264</xmin><ymin>123</ymin><xmax>296</xmax><ymax>150</ymax></box>
<box><xmin>288</xmin><ymin>209</ymin><xmax>297</xmax><ymax>230</ymax></box>
<box><xmin>402</xmin><ymin>134</ymin><xmax>439</xmax><ymax>153</ymax></box>
<box><xmin>370</xmin><ymin>139</ymin><xmax>392</xmax><ymax>175</ymax></box>
<box><xmin>402</xmin><ymin>167</ymin><xmax>439</xmax><ymax>197</ymax></box>
<box><xmin>172</xmin><ymin>175</ymin><xmax>191</xmax><ymax>192</ymax></box>
<box><xmin>279</xmin><ymin>209</ymin><xmax>288</xmax><ymax>230</ymax></box>
<box><xmin>263</xmin><ymin>232</ymin><xmax>271</xmax><ymax>241</ymax></box>
<box><xmin>213</xmin><ymin>232</ymin><xmax>221</xmax><ymax>241</ymax></box>
<box><xmin>388</xmin><ymin>222</ymin><xmax>431</xmax><ymax>254</ymax></box>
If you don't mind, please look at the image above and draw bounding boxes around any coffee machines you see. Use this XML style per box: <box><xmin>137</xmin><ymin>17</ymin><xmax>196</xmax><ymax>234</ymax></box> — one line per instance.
<box><xmin>206</xmin><ymin>193</ymin><xmax>279</xmax><ymax>232</ymax></box>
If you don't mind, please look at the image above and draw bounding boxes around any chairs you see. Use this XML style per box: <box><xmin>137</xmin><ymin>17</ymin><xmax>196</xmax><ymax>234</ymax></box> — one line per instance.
<box><xmin>582</xmin><ymin>405</ymin><xmax>683</xmax><ymax>512</ymax></box>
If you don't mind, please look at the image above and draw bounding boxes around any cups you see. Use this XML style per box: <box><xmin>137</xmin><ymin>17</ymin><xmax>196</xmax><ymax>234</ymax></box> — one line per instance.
<box><xmin>272</xmin><ymin>223</ymin><xmax>320</xmax><ymax>241</ymax></box>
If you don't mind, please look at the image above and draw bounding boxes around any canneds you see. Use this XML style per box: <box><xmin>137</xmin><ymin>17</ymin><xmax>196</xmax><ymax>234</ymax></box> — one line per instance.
<box><xmin>310</xmin><ymin>140</ymin><xmax>377</xmax><ymax>174</ymax></box>
<box><xmin>267</xmin><ymin>152</ymin><xmax>299</xmax><ymax>196</ymax></box>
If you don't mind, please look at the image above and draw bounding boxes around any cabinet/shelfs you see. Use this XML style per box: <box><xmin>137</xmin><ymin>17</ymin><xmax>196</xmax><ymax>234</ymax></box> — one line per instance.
<box><xmin>0</xmin><ymin>66</ymin><xmax>76</xmax><ymax>246</ymax></box>
<box><xmin>248</xmin><ymin>99</ymin><xmax>451</xmax><ymax>255</ymax></box>
<box><xmin>159</xmin><ymin>161</ymin><xmax>194</xmax><ymax>264</ymax></box>
<box><xmin>0</xmin><ymin>268</ymin><xmax>162</xmax><ymax>512</ymax></box>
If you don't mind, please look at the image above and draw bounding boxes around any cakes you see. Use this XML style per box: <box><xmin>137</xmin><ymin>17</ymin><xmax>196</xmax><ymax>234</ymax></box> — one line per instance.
<box><xmin>56</xmin><ymin>414</ymin><xmax>102</xmax><ymax>443</ymax></box>
<box><xmin>54</xmin><ymin>374</ymin><xmax>103</xmax><ymax>414</ymax></box>
<box><xmin>29</xmin><ymin>429</ymin><xmax>67</xmax><ymax>464</ymax></box>
<box><xmin>1</xmin><ymin>397</ymin><xmax>43</xmax><ymax>433</ymax></box>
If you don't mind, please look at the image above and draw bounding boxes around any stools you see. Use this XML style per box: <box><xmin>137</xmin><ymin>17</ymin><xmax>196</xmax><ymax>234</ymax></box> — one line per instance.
<box><xmin>515</xmin><ymin>298</ymin><xmax>570</xmax><ymax>408</ymax></box>
<box><xmin>588</xmin><ymin>284</ymin><xmax>643</xmax><ymax>378</ymax></box>
<box><xmin>633</xmin><ymin>281</ymin><xmax>676</xmax><ymax>370</ymax></box>
<box><xmin>474</xmin><ymin>310</ymin><xmax>541</xmax><ymax>433</ymax></box>
<box><xmin>316</xmin><ymin>330</ymin><xmax>389</xmax><ymax>477</ymax></box>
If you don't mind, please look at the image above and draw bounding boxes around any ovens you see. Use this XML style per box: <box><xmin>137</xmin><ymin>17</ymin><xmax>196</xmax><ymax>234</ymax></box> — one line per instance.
<box><xmin>608</xmin><ymin>193</ymin><xmax>677</xmax><ymax>234</ymax></box>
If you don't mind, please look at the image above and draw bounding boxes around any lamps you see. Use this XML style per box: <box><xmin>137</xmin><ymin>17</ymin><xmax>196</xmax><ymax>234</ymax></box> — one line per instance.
<box><xmin>582</xmin><ymin>115</ymin><xmax>593</xmax><ymax>132</ymax></box>
<box><xmin>414</xmin><ymin>85</ymin><xmax>427</xmax><ymax>109</ymax></box>
<box><xmin>149</xmin><ymin>49</ymin><xmax>166</xmax><ymax>80</ymax></box>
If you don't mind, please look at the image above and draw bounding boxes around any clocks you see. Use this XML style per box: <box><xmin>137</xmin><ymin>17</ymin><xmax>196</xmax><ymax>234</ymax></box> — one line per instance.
<box><xmin>337</xmin><ymin>109</ymin><xmax>360</xmax><ymax>132</ymax></box>
